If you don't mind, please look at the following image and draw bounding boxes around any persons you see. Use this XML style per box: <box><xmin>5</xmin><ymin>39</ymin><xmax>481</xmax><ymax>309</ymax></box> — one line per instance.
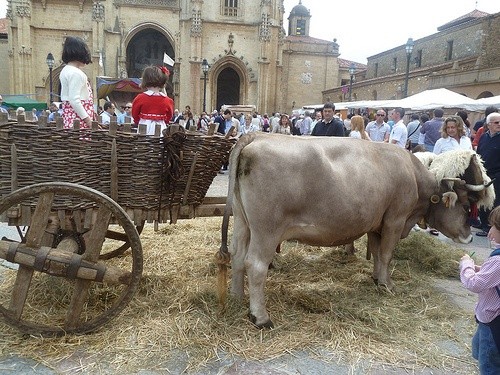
<box><xmin>365</xmin><ymin>106</ymin><xmax>500</xmax><ymax>235</ymax></box>
<box><xmin>214</xmin><ymin>102</ymin><xmax>372</xmax><ymax>174</ymax></box>
<box><xmin>59</xmin><ymin>36</ymin><xmax>98</xmax><ymax>141</ymax></box>
<box><xmin>99</xmin><ymin>101</ymin><xmax>225</xmax><ymax>135</ymax></box>
<box><xmin>0</xmin><ymin>95</ymin><xmax>63</xmax><ymax>120</ymax></box>
<box><xmin>132</xmin><ymin>65</ymin><xmax>175</xmax><ymax>137</ymax></box>
<box><xmin>458</xmin><ymin>205</ymin><xmax>500</xmax><ymax>375</ymax></box>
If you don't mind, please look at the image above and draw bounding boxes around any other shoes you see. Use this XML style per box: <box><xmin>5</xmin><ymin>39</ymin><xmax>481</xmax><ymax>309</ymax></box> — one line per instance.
<box><xmin>477</xmin><ymin>232</ymin><xmax>487</xmax><ymax>236</ymax></box>
<box><xmin>470</xmin><ymin>219</ymin><xmax>490</xmax><ymax>232</ymax></box>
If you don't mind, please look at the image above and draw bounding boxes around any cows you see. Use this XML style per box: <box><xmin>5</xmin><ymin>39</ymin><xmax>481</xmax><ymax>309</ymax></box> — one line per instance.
<box><xmin>210</xmin><ymin>129</ymin><xmax>497</xmax><ymax>332</ymax></box>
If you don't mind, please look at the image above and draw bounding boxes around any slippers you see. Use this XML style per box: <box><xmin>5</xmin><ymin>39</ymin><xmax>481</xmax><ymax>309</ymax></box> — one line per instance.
<box><xmin>430</xmin><ymin>230</ymin><xmax>438</xmax><ymax>235</ymax></box>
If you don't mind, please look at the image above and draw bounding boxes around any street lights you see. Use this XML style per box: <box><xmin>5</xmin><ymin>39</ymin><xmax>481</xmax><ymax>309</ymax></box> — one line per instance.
<box><xmin>202</xmin><ymin>58</ymin><xmax>209</xmax><ymax>112</ymax></box>
<box><xmin>348</xmin><ymin>63</ymin><xmax>355</xmax><ymax>102</ymax></box>
<box><xmin>403</xmin><ymin>38</ymin><xmax>414</xmax><ymax>99</ymax></box>
<box><xmin>46</xmin><ymin>53</ymin><xmax>55</xmax><ymax>104</ymax></box>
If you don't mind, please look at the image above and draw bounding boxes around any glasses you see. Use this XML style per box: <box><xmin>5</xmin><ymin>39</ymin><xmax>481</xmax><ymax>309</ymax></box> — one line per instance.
<box><xmin>377</xmin><ymin>114</ymin><xmax>384</xmax><ymax>116</ymax></box>
<box><xmin>125</xmin><ymin>107</ymin><xmax>132</xmax><ymax>109</ymax></box>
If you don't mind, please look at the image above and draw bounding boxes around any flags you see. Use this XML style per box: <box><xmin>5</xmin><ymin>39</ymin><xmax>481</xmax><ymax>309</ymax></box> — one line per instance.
<box><xmin>164</xmin><ymin>53</ymin><xmax>174</xmax><ymax>67</ymax></box>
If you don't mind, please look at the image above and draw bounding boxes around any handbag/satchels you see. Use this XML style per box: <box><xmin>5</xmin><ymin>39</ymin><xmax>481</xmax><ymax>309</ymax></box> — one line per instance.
<box><xmin>266</xmin><ymin>127</ymin><xmax>270</xmax><ymax>131</ymax></box>
<box><xmin>406</xmin><ymin>138</ymin><xmax>411</xmax><ymax>150</ymax></box>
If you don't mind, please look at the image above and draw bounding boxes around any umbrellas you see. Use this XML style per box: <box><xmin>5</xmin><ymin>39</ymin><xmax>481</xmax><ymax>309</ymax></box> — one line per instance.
<box><xmin>302</xmin><ymin>88</ymin><xmax>500</xmax><ymax>115</ymax></box>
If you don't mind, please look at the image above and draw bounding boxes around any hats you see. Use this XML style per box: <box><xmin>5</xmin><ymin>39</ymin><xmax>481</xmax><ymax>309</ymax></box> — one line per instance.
<box><xmin>421</xmin><ymin>114</ymin><xmax>428</xmax><ymax>123</ymax></box>
<box><xmin>486</xmin><ymin>106</ymin><xmax>498</xmax><ymax>117</ymax></box>
<box><xmin>473</xmin><ymin>121</ymin><xmax>483</xmax><ymax>131</ymax></box>
<box><xmin>224</xmin><ymin>109</ymin><xmax>230</xmax><ymax>114</ymax></box>
<box><xmin>410</xmin><ymin>115</ymin><xmax>419</xmax><ymax>119</ymax></box>
<box><xmin>214</xmin><ymin>110</ymin><xmax>217</xmax><ymax>113</ymax></box>
<box><xmin>433</xmin><ymin>108</ymin><xmax>444</xmax><ymax>117</ymax></box>
<box><xmin>457</xmin><ymin>111</ymin><xmax>467</xmax><ymax>120</ymax></box>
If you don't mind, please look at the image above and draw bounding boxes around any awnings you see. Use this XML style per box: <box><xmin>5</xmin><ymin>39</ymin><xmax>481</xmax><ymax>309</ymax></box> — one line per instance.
<box><xmin>96</xmin><ymin>76</ymin><xmax>146</xmax><ymax>98</ymax></box>
<box><xmin>1</xmin><ymin>94</ymin><xmax>47</xmax><ymax>111</ymax></box>
